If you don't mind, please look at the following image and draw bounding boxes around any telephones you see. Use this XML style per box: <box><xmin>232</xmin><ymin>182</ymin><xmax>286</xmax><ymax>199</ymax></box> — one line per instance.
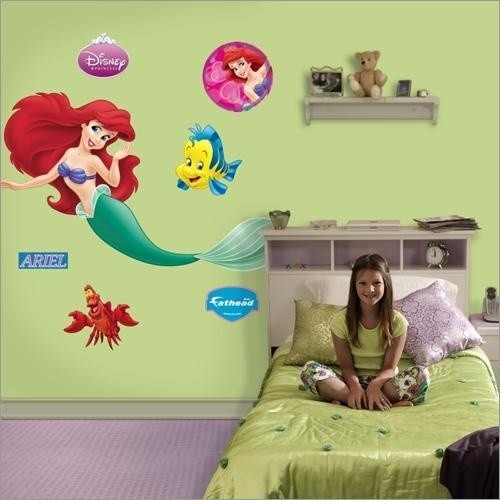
<box><xmin>482</xmin><ymin>288</ymin><xmax>500</xmax><ymax>323</ymax></box>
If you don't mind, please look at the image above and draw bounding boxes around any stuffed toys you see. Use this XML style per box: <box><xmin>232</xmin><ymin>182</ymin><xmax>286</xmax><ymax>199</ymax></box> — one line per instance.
<box><xmin>347</xmin><ymin>50</ymin><xmax>388</xmax><ymax>100</ymax></box>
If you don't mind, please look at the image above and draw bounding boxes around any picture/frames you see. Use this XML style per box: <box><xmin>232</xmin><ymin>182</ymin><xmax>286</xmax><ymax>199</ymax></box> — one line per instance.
<box><xmin>311</xmin><ymin>66</ymin><xmax>343</xmax><ymax>97</ymax></box>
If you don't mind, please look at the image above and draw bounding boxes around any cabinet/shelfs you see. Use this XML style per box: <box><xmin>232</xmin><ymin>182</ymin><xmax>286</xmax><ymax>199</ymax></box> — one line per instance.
<box><xmin>259</xmin><ymin>226</ymin><xmax>474</xmax><ymax>368</ymax></box>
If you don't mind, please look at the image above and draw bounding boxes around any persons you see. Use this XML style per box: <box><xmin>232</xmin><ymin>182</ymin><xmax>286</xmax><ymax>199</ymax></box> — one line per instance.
<box><xmin>299</xmin><ymin>252</ymin><xmax>431</xmax><ymax>412</ymax></box>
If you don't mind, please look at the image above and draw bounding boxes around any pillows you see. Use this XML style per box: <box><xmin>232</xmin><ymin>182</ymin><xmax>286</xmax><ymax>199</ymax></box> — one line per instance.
<box><xmin>281</xmin><ymin>275</ymin><xmax>483</xmax><ymax>366</ymax></box>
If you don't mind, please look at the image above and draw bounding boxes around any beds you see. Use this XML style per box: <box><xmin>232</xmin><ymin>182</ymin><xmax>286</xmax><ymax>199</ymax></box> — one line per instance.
<box><xmin>201</xmin><ymin>332</ymin><xmax>500</xmax><ymax>500</ymax></box>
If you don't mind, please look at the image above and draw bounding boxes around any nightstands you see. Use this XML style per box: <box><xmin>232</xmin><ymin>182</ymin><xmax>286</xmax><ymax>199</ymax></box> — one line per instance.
<box><xmin>469</xmin><ymin>313</ymin><xmax>500</xmax><ymax>396</ymax></box>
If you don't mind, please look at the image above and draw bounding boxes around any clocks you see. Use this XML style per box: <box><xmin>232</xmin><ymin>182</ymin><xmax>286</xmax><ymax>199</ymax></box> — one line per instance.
<box><xmin>425</xmin><ymin>242</ymin><xmax>450</xmax><ymax>270</ymax></box>
